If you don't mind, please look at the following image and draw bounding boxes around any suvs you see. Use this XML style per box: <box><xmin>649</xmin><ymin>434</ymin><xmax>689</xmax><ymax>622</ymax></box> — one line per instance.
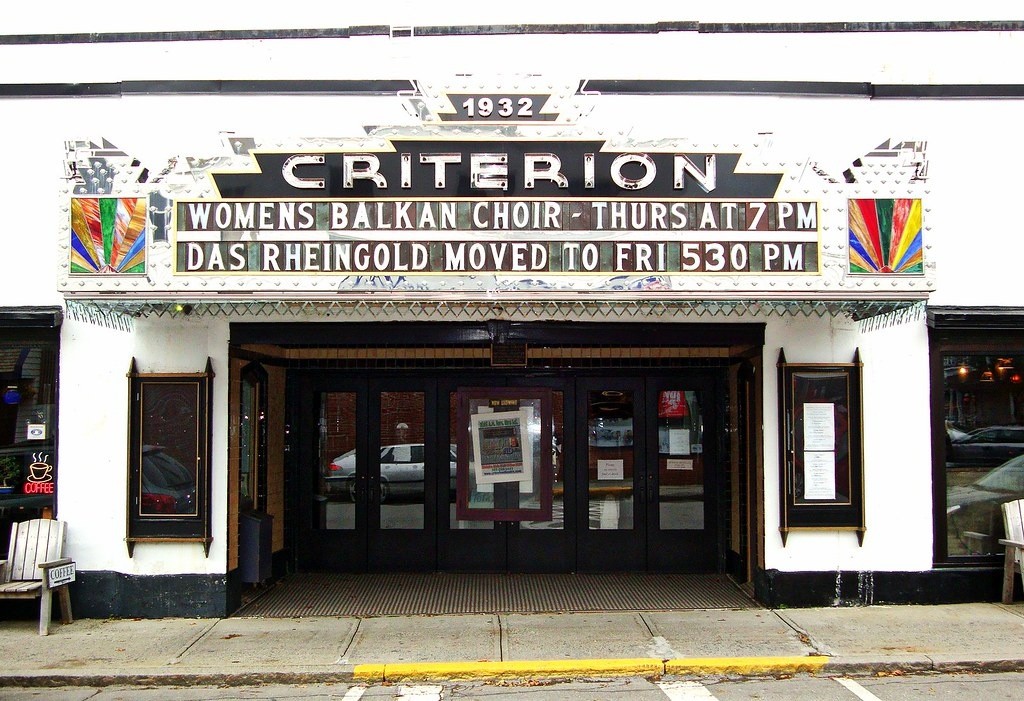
<box><xmin>0</xmin><ymin>445</ymin><xmax>196</xmax><ymax>561</ymax></box>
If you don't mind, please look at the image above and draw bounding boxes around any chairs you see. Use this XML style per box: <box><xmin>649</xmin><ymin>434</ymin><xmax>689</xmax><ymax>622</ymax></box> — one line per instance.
<box><xmin>0</xmin><ymin>518</ymin><xmax>76</xmax><ymax>636</ymax></box>
<box><xmin>963</xmin><ymin>500</ymin><xmax>1000</xmax><ymax>554</ymax></box>
<box><xmin>998</xmin><ymin>498</ymin><xmax>1024</xmax><ymax>604</ymax></box>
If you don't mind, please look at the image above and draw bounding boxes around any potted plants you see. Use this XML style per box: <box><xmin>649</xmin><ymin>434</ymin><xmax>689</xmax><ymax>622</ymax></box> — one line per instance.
<box><xmin>0</xmin><ymin>456</ymin><xmax>20</xmax><ymax>495</ymax></box>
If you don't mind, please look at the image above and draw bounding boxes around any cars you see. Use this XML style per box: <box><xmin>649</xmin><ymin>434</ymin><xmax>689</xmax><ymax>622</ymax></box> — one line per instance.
<box><xmin>327</xmin><ymin>442</ymin><xmax>479</xmax><ymax>503</ymax></box>
<box><xmin>947</xmin><ymin>424</ymin><xmax>970</xmax><ymax>442</ymax></box>
<box><xmin>946</xmin><ymin>425</ymin><xmax>1024</xmax><ymax>466</ymax></box>
<box><xmin>946</xmin><ymin>453</ymin><xmax>1024</xmax><ymax>557</ymax></box>
